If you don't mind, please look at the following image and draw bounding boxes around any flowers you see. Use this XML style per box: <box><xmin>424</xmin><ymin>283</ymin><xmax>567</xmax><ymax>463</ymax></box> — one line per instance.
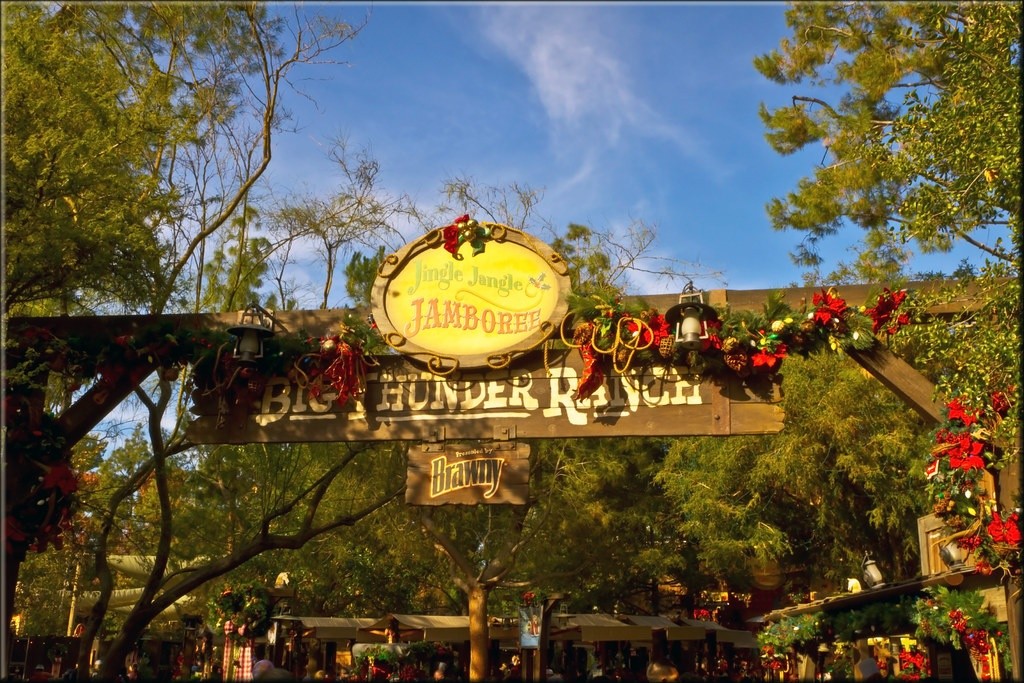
<box><xmin>718</xmin><ymin>285</ymin><xmax>913</xmax><ymax>368</ymax></box>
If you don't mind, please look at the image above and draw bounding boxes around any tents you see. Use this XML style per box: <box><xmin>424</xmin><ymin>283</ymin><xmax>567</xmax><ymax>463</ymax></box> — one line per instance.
<box><xmin>294</xmin><ymin>614</ymin><xmax>760</xmax><ymax>674</ymax></box>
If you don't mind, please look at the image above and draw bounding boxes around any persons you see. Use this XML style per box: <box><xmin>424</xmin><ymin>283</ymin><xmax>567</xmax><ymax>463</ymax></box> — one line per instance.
<box><xmin>172</xmin><ymin>650</ymin><xmax>185</xmax><ymax>679</ymax></box>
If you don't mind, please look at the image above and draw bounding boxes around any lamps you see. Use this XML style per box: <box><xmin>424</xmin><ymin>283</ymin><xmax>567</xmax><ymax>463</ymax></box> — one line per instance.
<box><xmin>861</xmin><ymin>550</ymin><xmax>888</xmax><ymax>591</ymax></box>
<box><xmin>664</xmin><ymin>279</ymin><xmax>719</xmax><ymax>350</ymax></box>
<box><xmin>939</xmin><ymin>542</ymin><xmax>965</xmax><ymax>571</ymax></box>
<box><xmin>226</xmin><ymin>301</ymin><xmax>292</xmax><ymax>364</ymax></box>
